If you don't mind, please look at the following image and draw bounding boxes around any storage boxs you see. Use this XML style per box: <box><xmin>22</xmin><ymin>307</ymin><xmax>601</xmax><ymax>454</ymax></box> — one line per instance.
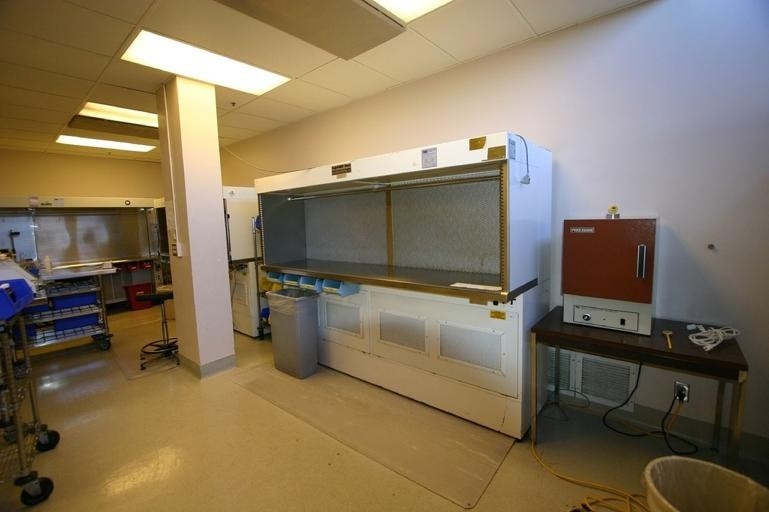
<box><xmin>122</xmin><ymin>282</ymin><xmax>154</xmax><ymax>310</ymax></box>
<box><xmin>0</xmin><ymin>278</ymin><xmax>99</xmax><ymax>341</ymax></box>
<box><xmin>267</xmin><ymin>271</ymin><xmax>359</xmax><ymax>298</ymax></box>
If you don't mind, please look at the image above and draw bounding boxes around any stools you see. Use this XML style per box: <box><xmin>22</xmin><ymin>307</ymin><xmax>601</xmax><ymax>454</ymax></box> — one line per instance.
<box><xmin>135</xmin><ymin>291</ymin><xmax>180</xmax><ymax>370</ymax></box>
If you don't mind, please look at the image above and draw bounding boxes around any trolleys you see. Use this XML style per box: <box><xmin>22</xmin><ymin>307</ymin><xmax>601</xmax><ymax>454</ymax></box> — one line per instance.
<box><xmin>7</xmin><ymin>266</ymin><xmax>120</xmax><ymax>380</ymax></box>
<box><xmin>0</xmin><ymin>287</ymin><xmax>60</xmax><ymax>505</ymax></box>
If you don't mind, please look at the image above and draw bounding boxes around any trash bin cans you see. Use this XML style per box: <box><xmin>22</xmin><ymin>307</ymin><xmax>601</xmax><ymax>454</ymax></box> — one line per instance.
<box><xmin>265</xmin><ymin>288</ymin><xmax>320</xmax><ymax>379</ymax></box>
<box><xmin>642</xmin><ymin>455</ymin><xmax>769</xmax><ymax>512</ymax></box>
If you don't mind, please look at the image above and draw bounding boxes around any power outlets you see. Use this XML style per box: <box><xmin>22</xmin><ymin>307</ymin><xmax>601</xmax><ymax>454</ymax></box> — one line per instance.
<box><xmin>673</xmin><ymin>381</ymin><xmax>689</xmax><ymax>402</ymax></box>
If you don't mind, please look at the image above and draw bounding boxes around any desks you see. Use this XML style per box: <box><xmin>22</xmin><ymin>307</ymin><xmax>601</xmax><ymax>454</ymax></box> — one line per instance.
<box><xmin>530</xmin><ymin>305</ymin><xmax>748</xmax><ymax>470</ymax></box>
